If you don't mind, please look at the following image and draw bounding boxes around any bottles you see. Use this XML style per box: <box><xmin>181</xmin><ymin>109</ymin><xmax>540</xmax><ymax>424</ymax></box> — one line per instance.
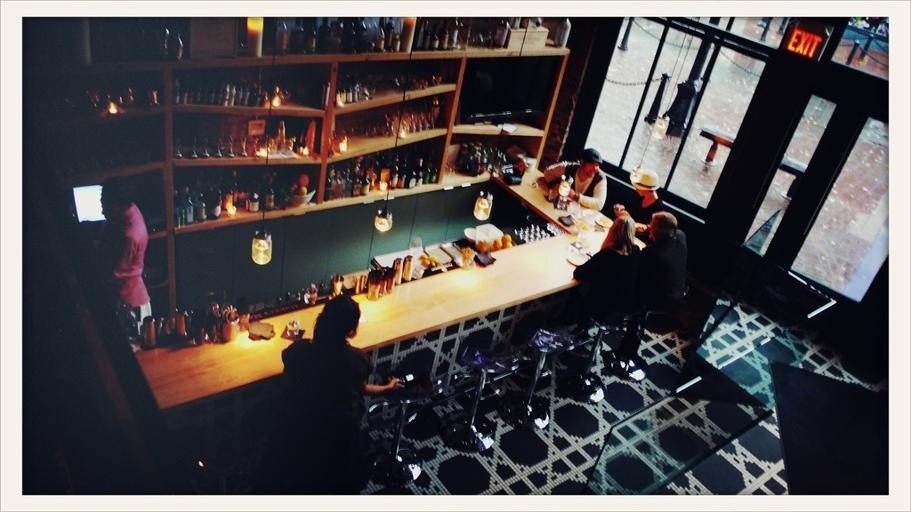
<box><xmin>331</xmin><ymin>251</ymin><xmax>415</xmax><ymax>302</ymax></box>
<box><xmin>548</xmin><ymin>174</ymin><xmax>573</xmax><ymax>210</ymax></box>
<box><xmin>169</xmin><ymin>71</ymin><xmax>438</xmax><ymax>226</ymax></box>
<box><xmin>120</xmin><ymin>294</ymin><xmax>239</xmax><ymax>352</ymax></box>
<box><xmin>461</xmin><ymin>140</ymin><xmax>508</xmax><ymax>178</ymax></box>
<box><xmin>270</xmin><ymin>18</ymin><xmax>570</xmax><ymax>52</ymax></box>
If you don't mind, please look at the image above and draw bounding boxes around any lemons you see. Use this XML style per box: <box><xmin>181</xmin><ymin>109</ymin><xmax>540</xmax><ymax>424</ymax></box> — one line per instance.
<box><xmin>422</xmin><ymin>257</ymin><xmax>435</xmax><ymax>267</ymax></box>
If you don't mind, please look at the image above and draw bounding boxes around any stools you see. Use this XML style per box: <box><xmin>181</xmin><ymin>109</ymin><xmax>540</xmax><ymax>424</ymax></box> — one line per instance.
<box><xmin>440</xmin><ymin>350</ymin><xmax>538</xmax><ymax>454</ymax></box>
<box><xmin>365</xmin><ymin>367</ymin><xmax>438</xmax><ymax>486</ymax></box>
<box><xmin>557</xmin><ymin>310</ymin><xmax>628</xmax><ymax>403</ymax></box>
<box><xmin>495</xmin><ymin>320</ymin><xmax>595</xmax><ymax>432</ymax></box>
<box><xmin>608</xmin><ymin>310</ymin><xmax>661</xmax><ymax>382</ymax></box>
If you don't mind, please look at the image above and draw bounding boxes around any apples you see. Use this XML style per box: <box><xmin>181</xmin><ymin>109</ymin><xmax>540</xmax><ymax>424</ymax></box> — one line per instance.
<box><xmin>298</xmin><ymin>186</ymin><xmax>307</xmax><ymax>196</ymax></box>
<box><xmin>297</xmin><ymin>174</ymin><xmax>311</xmax><ymax>187</ymax></box>
<box><xmin>288</xmin><ymin>181</ymin><xmax>297</xmax><ymax>193</ymax></box>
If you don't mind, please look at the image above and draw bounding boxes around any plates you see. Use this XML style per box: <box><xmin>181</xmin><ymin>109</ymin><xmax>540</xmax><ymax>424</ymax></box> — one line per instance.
<box><xmin>567</xmin><ymin>254</ymin><xmax>589</xmax><ymax>267</ymax></box>
<box><xmin>595</xmin><ymin>217</ymin><xmax>612</xmax><ymax>229</ymax></box>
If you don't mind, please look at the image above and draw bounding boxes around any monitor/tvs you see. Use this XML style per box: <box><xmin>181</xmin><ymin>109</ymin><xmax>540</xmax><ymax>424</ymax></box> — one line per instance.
<box><xmin>69</xmin><ymin>184</ymin><xmax>106</xmax><ymax>224</ymax></box>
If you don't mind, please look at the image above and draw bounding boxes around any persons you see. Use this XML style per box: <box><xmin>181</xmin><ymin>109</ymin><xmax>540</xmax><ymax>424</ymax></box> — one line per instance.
<box><xmin>280</xmin><ymin>295</ymin><xmax>406</xmax><ymax>493</ymax></box>
<box><xmin>636</xmin><ymin>211</ymin><xmax>688</xmax><ymax>308</ymax></box>
<box><xmin>84</xmin><ymin>177</ymin><xmax>152</xmax><ymax>334</ymax></box>
<box><xmin>573</xmin><ymin>215</ymin><xmax>643</xmax><ymax>330</ymax></box>
<box><xmin>544</xmin><ymin>148</ymin><xmax>607</xmax><ymax>211</ymax></box>
<box><xmin>614</xmin><ymin>169</ymin><xmax>664</xmax><ymax>245</ymax></box>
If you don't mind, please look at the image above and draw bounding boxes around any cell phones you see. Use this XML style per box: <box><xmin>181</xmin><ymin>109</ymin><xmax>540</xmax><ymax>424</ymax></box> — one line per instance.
<box><xmin>398</xmin><ymin>372</ymin><xmax>417</xmax><ymax>384</ymax></box>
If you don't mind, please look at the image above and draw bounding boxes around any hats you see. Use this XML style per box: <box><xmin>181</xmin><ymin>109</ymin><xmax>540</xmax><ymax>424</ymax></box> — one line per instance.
<box><xmin>581</xmin><ymin>147</ymin><xmax>604</xmax><ymax>166</ymax></box>
<box><xmin>629</xmin><ymin>168</ymin><xmax>662</xmax><ymax>192</ymax></box>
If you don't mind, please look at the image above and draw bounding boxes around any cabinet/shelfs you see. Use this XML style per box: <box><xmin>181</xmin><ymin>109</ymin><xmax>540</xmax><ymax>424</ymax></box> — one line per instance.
<box><xmin>23</xmin><ymin>39</ymin><xmax>571</xmax><ymax>317</ymax></box>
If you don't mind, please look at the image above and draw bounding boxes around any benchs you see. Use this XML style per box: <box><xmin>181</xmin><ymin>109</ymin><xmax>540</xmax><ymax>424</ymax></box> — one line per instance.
<box><xmin>701</xmin><ymin>127</ymin><xmax>809</xmax><ymax>199</ymax></box>
